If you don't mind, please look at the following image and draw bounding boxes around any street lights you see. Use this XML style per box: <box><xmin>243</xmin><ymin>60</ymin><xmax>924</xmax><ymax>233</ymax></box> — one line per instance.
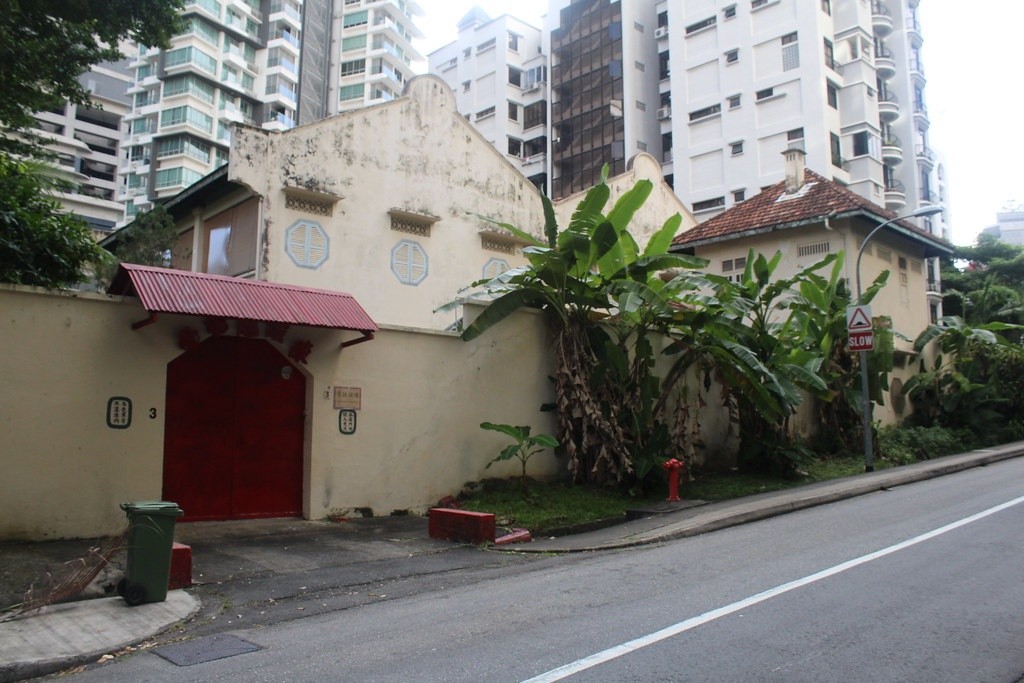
<box><xmin>856</xmin><ymin>205</ymin><xmax>942</xmax><ymax>473</ymax></box>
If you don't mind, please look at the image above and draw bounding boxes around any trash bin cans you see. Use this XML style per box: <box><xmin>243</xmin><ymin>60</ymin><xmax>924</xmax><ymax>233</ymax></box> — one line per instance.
<box><xmin>117</xmin><ymin>500</ymin><xmax>185</xmax><ymax>607</ymax></box>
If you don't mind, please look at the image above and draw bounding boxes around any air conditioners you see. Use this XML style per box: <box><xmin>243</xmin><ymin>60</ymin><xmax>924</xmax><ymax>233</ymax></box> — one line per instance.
<box><xmin>654</xmin><ymin>26</ymin><xmax>666</xmax><ymax>39</ymax></box>
<box><xmin>656</xmin><ymin>108</ymin><xmax>667</xmax><ymax>120</ymax></box>
<box><xmin>529</xmin><ymin>81</ymin><xmax>538</xmax><ymax>92</ymax></box>
